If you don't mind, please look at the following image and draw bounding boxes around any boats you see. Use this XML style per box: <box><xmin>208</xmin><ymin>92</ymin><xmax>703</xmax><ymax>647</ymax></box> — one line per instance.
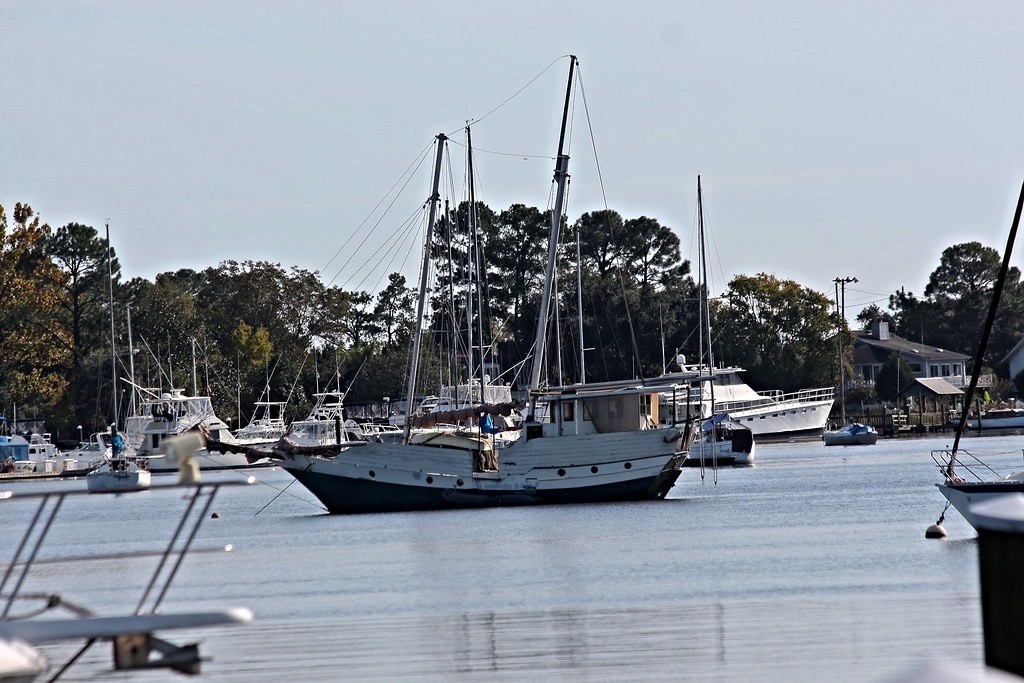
<box><xmin>951</xmin><ymin>406</ymin><xmax>1024</xmax><ymax>429</ymax></box>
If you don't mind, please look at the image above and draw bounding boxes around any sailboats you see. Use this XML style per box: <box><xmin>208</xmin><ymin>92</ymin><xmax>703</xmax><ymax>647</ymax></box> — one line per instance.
<box><xmin>0</xmin><ymin>54</ymin><xmax>835</xmax><ymax>516</ymax></box>
<box><xmin>820</xmin><ymin>283</ymin><xmax>879</xmax><ymax>446</ymax></box>
<box><xmin>927</xmin><ymin>172</ymin><xmax>1024</xmax><ymax>534</ymax></box>
